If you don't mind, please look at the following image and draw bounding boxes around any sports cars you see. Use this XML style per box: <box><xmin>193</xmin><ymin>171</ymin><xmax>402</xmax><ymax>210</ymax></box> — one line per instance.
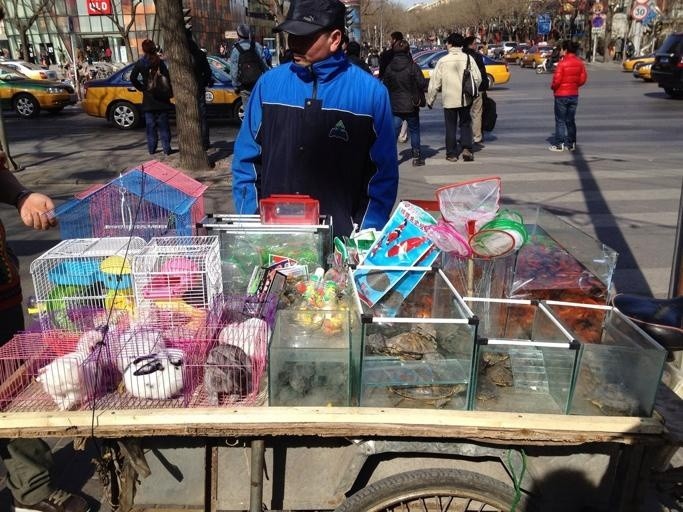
<box><xmin>0</xmin><ymin>292</ymin><xmax>683</xmax><ymax>512</ymax></box>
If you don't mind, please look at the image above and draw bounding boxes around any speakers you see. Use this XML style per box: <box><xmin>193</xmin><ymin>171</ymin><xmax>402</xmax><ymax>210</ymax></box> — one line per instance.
<box><xmin>411</xmin><ymin>147</ymin><xmax>425</xmax><ymax>166</ymax></box>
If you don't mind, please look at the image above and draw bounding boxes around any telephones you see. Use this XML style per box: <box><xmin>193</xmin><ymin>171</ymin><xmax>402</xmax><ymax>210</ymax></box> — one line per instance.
<box><xmin>630</xmin><ymin>5</ymin><xmax>647</xmax><ymax>20</ymax></box>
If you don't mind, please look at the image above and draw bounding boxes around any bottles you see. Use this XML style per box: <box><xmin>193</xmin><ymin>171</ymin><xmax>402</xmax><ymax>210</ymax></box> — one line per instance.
<box><xmin>272</xmin><ymin>0</ymin><xmax>347</xmax><ymax>36</ymax></box>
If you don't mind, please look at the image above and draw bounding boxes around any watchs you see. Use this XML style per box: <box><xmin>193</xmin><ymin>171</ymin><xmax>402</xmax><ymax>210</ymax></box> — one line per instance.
<box><xmin>536</xmin><ymin>53</ymin><xmax>558</xmax><ymax>74</ymax></box>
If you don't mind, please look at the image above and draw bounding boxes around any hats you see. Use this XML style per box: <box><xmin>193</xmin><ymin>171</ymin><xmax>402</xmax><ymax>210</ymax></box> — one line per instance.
<box><xmin>206</xmin><ymin>55</ymin><xmax>230</xmax><ymax>74</ymax></box>
<box><xmin>477</xmin><ymin>40</ymin><xmax>556</xmax><ymax>68</ymax></box>
<box><xmin>80</xmin><ymin>54</ymin><xmax>245</xmax><ymax>129</ymax></box>
<box><xmin>0</xmin><ymin>63</ymin><xmax>78</xmax><ymax>119</ymax></box>
<box><xmin>623</xmin><ymin>51</ymin><xmax>655</xmax><ymax>71</ymax></box>
<box><xmin>633</xmin><ymin>62</ymin><xmax>655</xmax><ymax>82</ymax></box>
<box><xmin>372</xmin><ymin>46</ymin><xmax>512</xmax><ymax>92</ymax></box>
<box><xmin>0</xmin><ymin>59</ymin><xmax>57</xmax><ymax>80</ymax></box>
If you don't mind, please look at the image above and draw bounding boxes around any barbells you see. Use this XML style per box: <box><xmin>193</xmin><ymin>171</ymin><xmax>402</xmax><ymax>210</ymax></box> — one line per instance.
<box><xmin>400</xmin><ymin>221</ymin><xmax>609</xmax><ymax>344</ymax></box>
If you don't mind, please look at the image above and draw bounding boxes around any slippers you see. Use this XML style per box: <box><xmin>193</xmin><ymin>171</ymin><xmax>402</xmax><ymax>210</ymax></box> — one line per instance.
<box><xmin>234</xmin><ymin>38</ymin><xmax>266</xmax><ymax>91</ymax></box>
<box><xmin>482</xmin><ymin>93</ymin><xmax>497</xmax><ymax>132</ymax></box>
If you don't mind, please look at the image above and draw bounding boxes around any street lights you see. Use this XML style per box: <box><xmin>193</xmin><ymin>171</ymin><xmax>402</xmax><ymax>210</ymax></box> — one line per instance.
<box><xmin>148</xmin><ymin>68</ymin><xmax>173</xmax><ymax>98</ymax></box>
<box><xmin>463</xmin><ymin>69</ymin><xmax>478</xmax><ymax>97</ymax></box>
<box><xmin>411</xmin><ymin>82</ymin><xmax>426</xmax><ymax>107</ymax></box>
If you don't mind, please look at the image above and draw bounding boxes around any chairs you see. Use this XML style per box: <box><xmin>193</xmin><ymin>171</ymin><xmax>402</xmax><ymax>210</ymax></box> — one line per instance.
<box><xmin>548</xmin><ymin>142</ymin><xmax>565</xmax><ymax>152</ymax></box>
<box><xmin>462</xmin><ymin>149</ymin><xmax>475</xmax><ymax>161</ymax></box>
<box><xmin>564</xmin><ymin>142</ymin><xmax>577</xmax><ymax>150</ymax></box>
<box><xmin>12</xmin><ymin>488</ymin><xmax>90</xmax><ymax>512</ymax></box>
<box><xmin>445</xmin><ymin>154</ymin><xmax>459</xmax><ymax>162</ymax></box>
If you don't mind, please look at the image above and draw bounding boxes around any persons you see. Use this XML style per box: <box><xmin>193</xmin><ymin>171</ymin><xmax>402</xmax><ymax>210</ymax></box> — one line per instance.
<box><xmin>229</xmin><ymin>1</ymin><xmax>398</xmax><ymax>242</ymax></box>
<box><xmin>228</xmin><ymin>21</ymin><xmax>271</xmax><ymax>113</ymax></box>
<box><xmin>425</xmin><ymin>34</ymin><xmax>482</xmax><ymax>163</ymax></box>
<box><xmin>0</xmin><ymin>140</ymin><xmax>99</xmax><ymax>509</ymax></box>
<box><xmin>460</xmin><ymin>32</ymin><xmax>502</xmax><ymax>150</ymax></box>
<box><xmin>129</xmin><ymin>39</ymin><xmax>173</xmax><ymax>155</ymax></box>
<box><xmin>549</xmin><ymin>39</ymin><xmax>588</xmax><ymax>152</ymax></box>
<box><xmin>383</xmin><ymin>41</ymin><xmax>425</xmax><ymax>166</ymax></box>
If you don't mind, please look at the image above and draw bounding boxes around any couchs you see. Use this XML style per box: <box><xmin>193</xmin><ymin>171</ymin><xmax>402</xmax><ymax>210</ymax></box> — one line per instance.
<box><xmin>344</xmin><ymin>6</ymin><xmax>354</xmax><ymax>30</ymax></box>
<box><xmin>182</xmin><ymin>7</ymin><xmax>193</xmax><ymax>30</ymax></box>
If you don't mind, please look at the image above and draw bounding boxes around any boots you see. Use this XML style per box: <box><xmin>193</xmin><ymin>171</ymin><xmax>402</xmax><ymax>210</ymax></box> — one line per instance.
<box><xmin>652</xmin><ymin>31</ymin><xmax>682</xmax><ymax>97</ymax></box>
